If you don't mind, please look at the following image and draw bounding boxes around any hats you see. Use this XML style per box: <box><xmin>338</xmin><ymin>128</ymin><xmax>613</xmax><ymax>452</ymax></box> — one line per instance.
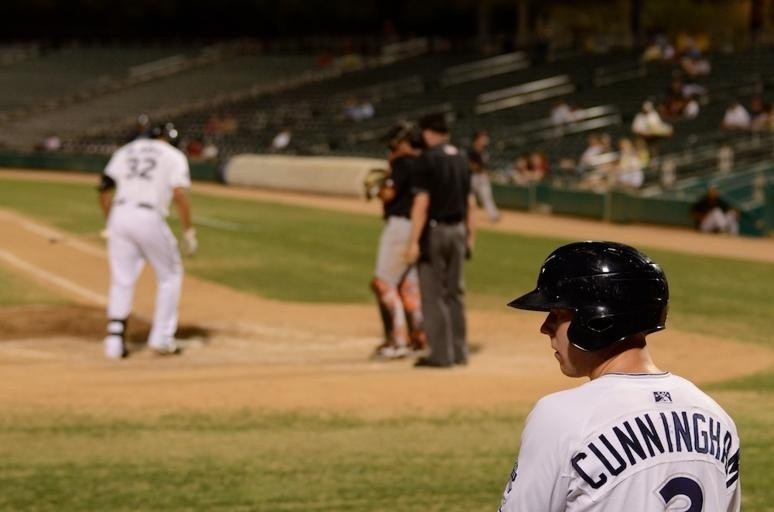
<box><xmin>381</xmin><ymin>104</ymin><xmax>459</xmax><ymax>153</ymax></box>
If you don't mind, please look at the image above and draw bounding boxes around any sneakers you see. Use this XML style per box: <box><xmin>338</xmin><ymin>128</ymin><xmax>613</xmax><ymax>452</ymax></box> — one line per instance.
<box><xmin>376</xmin><ymin>331</ymin><xmax>445</xmax><ymax>367</ymax></box>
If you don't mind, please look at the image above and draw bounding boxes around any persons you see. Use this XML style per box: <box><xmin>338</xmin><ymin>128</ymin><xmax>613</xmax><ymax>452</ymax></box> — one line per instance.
<box><xmin>572</xmin><ymin>32</ymin><xmax>767</xmax><ymax>193</ymax></box>
<box><xmin>369</xmin><ymin>129</ymin><xmax>429</xmax><ymax>364</ymax></box>
<box><xmin>686</xmin><ymin>184</ymin><xmax>747</xmax><ymax>237</ymax></box>
<box><xmin>493</xmin><ymin>241</ymin><xmax>743</xmax><ymax>512</ymax></box>
<box><xmin>549</xmin><ymin>96</ymin><xmax>580</xmax><ymax>137</ymax></box>
<box><xmin>138</xmin><ymin>112</ymin><xmax>235</xmax><ymax>160</ymax></box>
<box><xmin>465</xmin><ymin>130</ymin><xmax>501</xmax><ymax>221</ymax></box>
<box><xmin>95</xmin><ymin>118</ymin><xmax>199</xmax><ymax>358</ymax></box>
<box><xmin>504</xmin><ymin>153</ymin><xmax>548</xmax><ymax>188</ymax></box>
<box><xmin>336</xmin><ymin>96</ymin><xmax>376</xmax><ymax>122</ymax></box>
<box><xmin>404</xmin><ymin>110</ymin><xmax>473</xmax><ymax>367</ymax></box>
<box><xmin>38</xmin><ymin>132</ymin><xmax>64</xmax><ymax>151</ymax></box>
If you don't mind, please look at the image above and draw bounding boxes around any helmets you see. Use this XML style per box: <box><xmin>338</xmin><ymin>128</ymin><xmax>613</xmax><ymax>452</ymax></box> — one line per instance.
<box><xmin>506</xmin><ymin>240</ymin><xmax>669</xmax><ymax>353</ymax></box>
<box><xmin>147</xmin><ymin>120</ymin><xmax>179</xmax><ymax>147</ymax></box>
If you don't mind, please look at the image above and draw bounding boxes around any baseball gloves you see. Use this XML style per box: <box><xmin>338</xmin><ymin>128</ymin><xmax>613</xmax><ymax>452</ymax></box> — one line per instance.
<box><xmin>363</xmin><ymin>168</ymin><xmax>390</xmax><ymax>201</ymax></box>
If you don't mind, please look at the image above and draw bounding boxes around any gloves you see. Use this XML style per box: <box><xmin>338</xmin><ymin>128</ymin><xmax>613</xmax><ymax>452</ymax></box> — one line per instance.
<box><xmin>185</xmin><ymin>229</ymin><xmax>197</xmax><ymax>257</ymax></box>
